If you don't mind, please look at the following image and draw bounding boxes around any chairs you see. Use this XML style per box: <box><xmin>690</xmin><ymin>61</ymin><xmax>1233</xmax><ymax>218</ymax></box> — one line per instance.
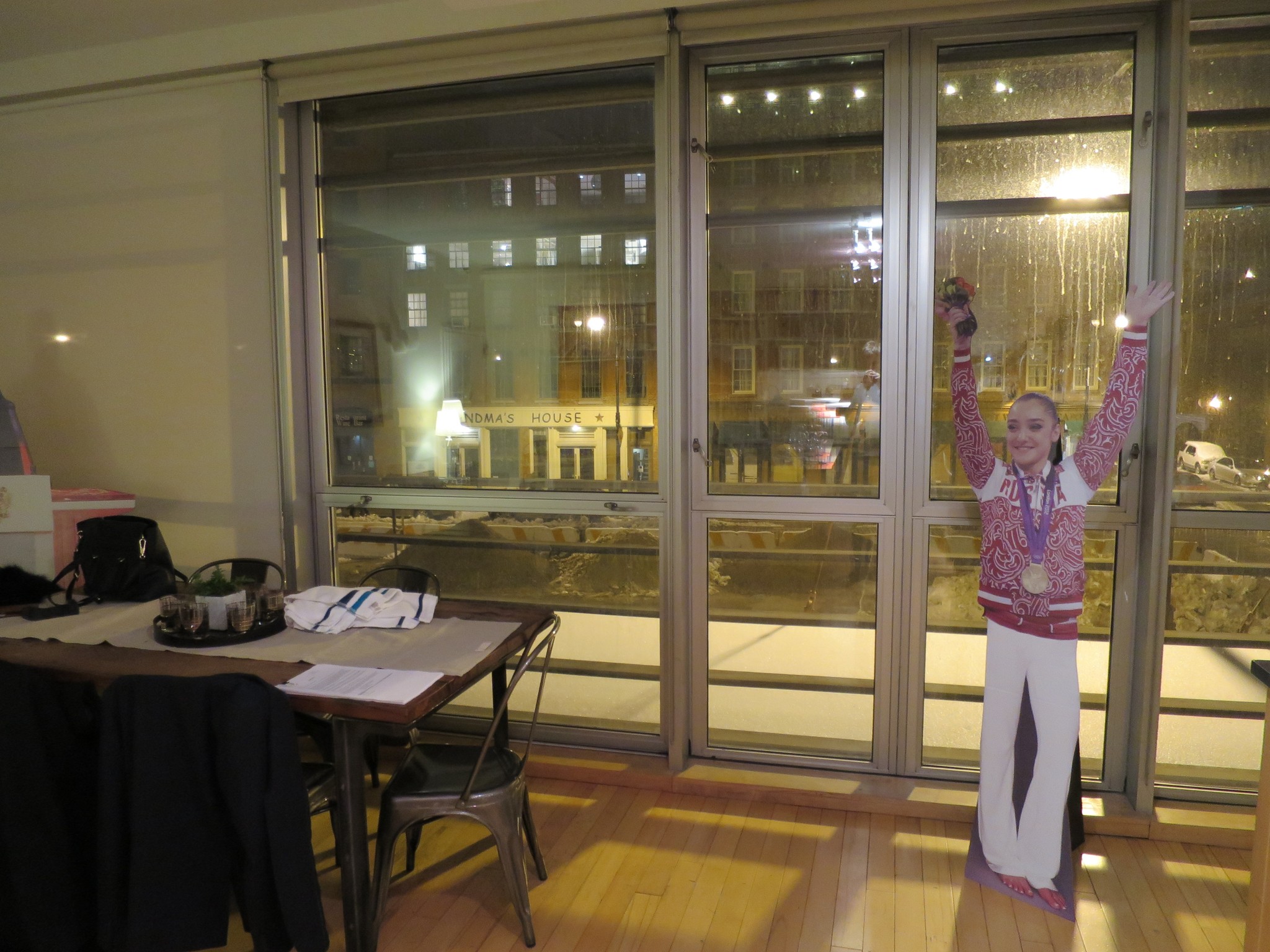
<box><xmin>355</xmin><ymin>564</ymin><xmax>442</xmax><ymax>602</ymax></box>
<box><xmin>185</xmin><ymin>557</ymin><xmax>287</xmax><ymax>598</ymax></box>
<box><xmin>366</xmin><ymin>609</ymin><xmax>562</xmax><ymax>952</ymax></box>
<box><xmin>304</xmin><ymin>759</ymin><xmax>345</xmax><ymax>857</ymax></box>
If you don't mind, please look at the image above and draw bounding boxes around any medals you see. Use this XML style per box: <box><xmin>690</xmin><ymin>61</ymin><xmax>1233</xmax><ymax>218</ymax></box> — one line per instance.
<box><xmin>1019</xmin><ymin>553</ymin><xmax>1055</xmax><ymax>594</ymax></box>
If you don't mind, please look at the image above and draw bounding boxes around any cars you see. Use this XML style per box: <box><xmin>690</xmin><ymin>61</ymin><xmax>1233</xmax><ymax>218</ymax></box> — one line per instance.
<box><xmin>1208</xmin><ymin>457</ymin><xmax>1268</xmax><ymax>489</ymax></box>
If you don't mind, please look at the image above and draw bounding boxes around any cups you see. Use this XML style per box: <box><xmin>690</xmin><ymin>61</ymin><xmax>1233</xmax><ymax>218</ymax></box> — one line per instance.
<box><xmin>178</xmin><ymin>602</ymin><xmax>210</xmax><ymax>639</ymax></box>
<box><xmin>226</xmin><ymin>599</ymin><xmax>256</xmax><ymax>632</ymax></box>
<box><xmin>157</xmin><ymin>593</ymin><xmax>189</xmax><ymax>633</ymax></box>
<box><xmin>257</xmin><ymin>588</ymin><xmax>285</xmax><ymax>627</ymax></box>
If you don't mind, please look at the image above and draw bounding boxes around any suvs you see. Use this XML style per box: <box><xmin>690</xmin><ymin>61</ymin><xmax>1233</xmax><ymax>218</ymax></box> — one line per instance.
<box><xmin>1176</xmin><ymin>440</ymin><xmax>1225</xmax><ymax>474</ymax></box>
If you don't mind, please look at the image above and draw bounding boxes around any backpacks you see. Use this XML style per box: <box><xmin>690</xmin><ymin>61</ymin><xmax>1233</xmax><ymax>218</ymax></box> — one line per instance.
<box><xmin>46</xmin><ymin>514</ymin><xmax>189</xmax><ymax>609</ymax></box>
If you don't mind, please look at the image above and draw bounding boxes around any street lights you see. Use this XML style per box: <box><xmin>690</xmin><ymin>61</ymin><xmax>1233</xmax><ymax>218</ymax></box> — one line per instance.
<box><xmin>586</xmin><ymin>315</ymin><xmax>623</xmax><ymax>481</ymax></box>
<box><xmin>1080</xmin><ymin>311</ymin><xmax>1129</xmax><ymax>435</ymax></box>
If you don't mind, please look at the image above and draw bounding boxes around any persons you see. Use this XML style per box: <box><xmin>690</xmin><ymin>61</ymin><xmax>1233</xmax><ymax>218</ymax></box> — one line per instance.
<box><xmin>943</xmin><ymin>274</ymin><xmax>1177</xmax><ymax>911</ymax></box>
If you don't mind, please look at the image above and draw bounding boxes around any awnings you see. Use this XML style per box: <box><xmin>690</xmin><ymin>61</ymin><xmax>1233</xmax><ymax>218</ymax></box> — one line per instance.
<box><xmin>709</xmin><ymin>420</ymin><xmax>1091</xmax><ymax>439</ymax></box>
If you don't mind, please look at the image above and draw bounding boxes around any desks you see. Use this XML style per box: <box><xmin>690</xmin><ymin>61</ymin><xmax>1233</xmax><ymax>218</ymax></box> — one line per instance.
<box><xmin>0</xmin><ymin>580</ymin><xmax>554</xmax><ymax>952</ymax></box>
